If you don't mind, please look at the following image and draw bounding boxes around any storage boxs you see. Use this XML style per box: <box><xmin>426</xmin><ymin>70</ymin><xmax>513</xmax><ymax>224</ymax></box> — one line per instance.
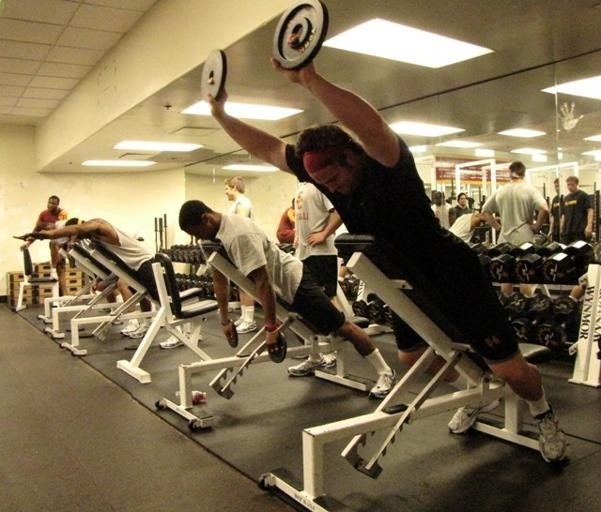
<box><xmin>8</xmin><ymin>262</ymin><xmax>84</xmax><ymax>306</ymax></box>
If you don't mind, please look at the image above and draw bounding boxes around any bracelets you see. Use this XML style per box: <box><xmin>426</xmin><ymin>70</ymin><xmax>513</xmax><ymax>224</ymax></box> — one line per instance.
<box><xmin>221</xmin><ymin>314</ymin><xmax>230</xmax><ymax>320</ymax></box>
<box><xmin>266</xmin><ymin>319</ymin><xmax>279</xmax><ymax>335</ymax></box>
<box><xmin>222</xmin><ymin>320</ymin><xmax>230</xmax><ymax>326</ymax></box>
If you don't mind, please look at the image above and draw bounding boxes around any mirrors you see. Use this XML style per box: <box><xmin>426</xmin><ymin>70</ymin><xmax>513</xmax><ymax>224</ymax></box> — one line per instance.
<box><xmin>185</xmin><ymin>47</ymin><xmax>600</xmax><ymax>254</ymax></box>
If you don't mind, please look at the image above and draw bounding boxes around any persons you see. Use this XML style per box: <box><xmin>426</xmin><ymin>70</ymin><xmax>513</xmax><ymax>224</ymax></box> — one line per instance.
<box><xmin>224</xmin><ymin>177</ymin><xmax>259</xmax><ymax>333</ymax></box>
<box><xmin>14</xmin><ymin>218</ymin><xmax>186</xmax><ymax>348</ymax></box>
<box><xmin>208</xmin><ymin>34</ymin><xmax>565</xmax><ymax>463</ymax></box>
<box><xmin>482</xmin><ymin>161</ymin><xmax>550</xmax><ymax>297</ymax></box>
<box><xmin>431</xmin><ymin>190</ymin><xmax>453</xmax><ymax>229</ymax></box>
<box><xmin>561</xmin><ymin>177</ymin><xmax>594</xmax><ymax>241</ymax></box>
<box><xmin>22</xmin><ymin>196</ymin><xmax>69</xmax><ymax>296</ymax></box>
<box><xmin>288</xmin><ymin>183</ymin><xmax>343</xmax><ymax>376</ymax></box>
<box><xmin>449</xmin><ymin>210</ymin><xmax>501</xmax><ymax>245</ymax></box>
<box><xmin>450</xmin><ymin>192</ymin><xmax>472</xmax><ymax>224</ymax></box>
<box><xmin>180</xmin><ymin>200</ymin><xmax>398</xmax><ymax>398</ymax></box>
<box><xmin>277</xmin><ymin>198</ymin><xmax>296</xmax><ymax>243</ymax></box>
<box><xmin>36</xmin><ymin>220</ymin><xmax>141</xmax><ymax>337</ymax></box>
<box><xmin>546</xmin><ymin>178</ymin><xmax>566</xmax><ymax>241</ymax></box>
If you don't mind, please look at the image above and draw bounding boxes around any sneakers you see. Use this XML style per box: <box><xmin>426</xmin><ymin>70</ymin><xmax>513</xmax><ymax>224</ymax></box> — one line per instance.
<box><xmin>533</xmin><ymin>401</ymin><xmax>566</xmax><ymax>464</ymax></box>
<box><xmin>234</xmin><ymin>316</ymin><xmax>244</xmax><ymax>326</ymax></box>
<box><xmin>447</xmin><ymin>398</ymin><xmax>500</xmax><ymax>434</ymax></box>
<box><xmin>287</xmin><ymin>355</ymin><xmax>327</xmax><ymax>376</ymax></box>
<box><xmin>236</xmin><ymin>320</ymin><xmax>256</xmax><ymax>334</ymax></box>
<box><xmin>160</xmin><ymin>330</ymin><xmax>184</xmax><ymax>349</ymax></box>
<box><xmin>128</xmin><ymin>322</ymin><xmax>152</xmax><ymax>338</ymax></box>
<box><xmin>317</xmin><ymin>342</ymin><xmax>336</xmax><ymax>368</ymax></box>
<box><xmin>369</xmin><ymin>369</ymin><xmax>396</xmax><ymax>400</ymax></box>
<box><xmin>120</xmin><ymin>321</ymin><xmax>139</xmax><ymax>336</ymax></box>
